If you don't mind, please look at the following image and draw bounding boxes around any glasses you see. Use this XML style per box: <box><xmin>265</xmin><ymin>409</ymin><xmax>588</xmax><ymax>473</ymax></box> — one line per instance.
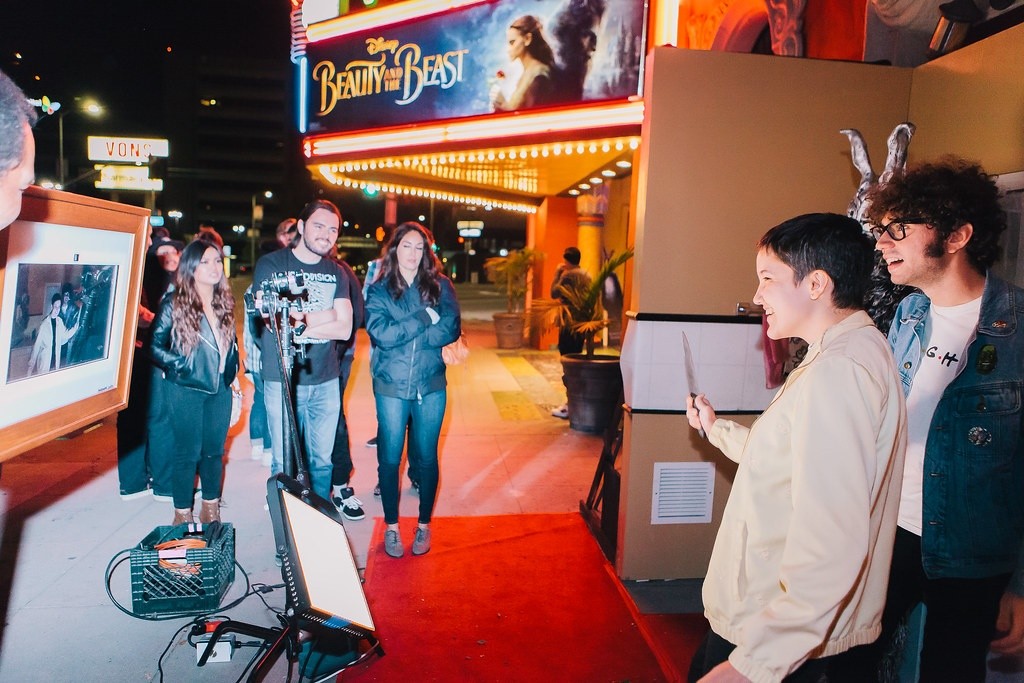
<box><xmin>870</xmin><ymin>217</ymin><xmax>937</xmax><ymax>242</ymax></box>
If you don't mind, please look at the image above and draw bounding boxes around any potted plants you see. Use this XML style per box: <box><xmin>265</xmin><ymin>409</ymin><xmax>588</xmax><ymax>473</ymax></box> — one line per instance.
<box><xmin>526</xmin><ymin>245</ymin><xmax>634</xmax><ymax>433</ymax></box>
<box><xmin>483</xmin><ymin>247</ymin><xmax>531</xmax><ymax>348</ymax></box>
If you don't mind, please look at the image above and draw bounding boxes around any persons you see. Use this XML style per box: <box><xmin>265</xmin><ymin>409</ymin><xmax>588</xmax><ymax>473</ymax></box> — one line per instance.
<box><xmin>551</xmin><ymin>246</ymin><xmax>593</xmax><ymax>419</ymax></box>
<box><xmin>27</xmin><ymin>283</ymin><xmax>81</xmax><ymax>377</ymax></box>
<box><xmin>686</xmin><ymin>212</ymin><xmax>908</xmax><ymax>683</ymax></box>
<box><xmin>0</xmin><ymin>68</ymin><xmax>36</xmax><ymax>233</ymax></box>
<box><xmin>870</xmin><ymin>153</ymin><xmax>1024</xmax><ymax>683</ymax></box>
<box><xmin>117</xmin><ymin>198</ymin><xmax>461</xmax><ymax>559</ymax></box>
<box><xmin>490</xmin><ymin>14</ymin><xmax>556</xmax><ymax>112</ymax></box>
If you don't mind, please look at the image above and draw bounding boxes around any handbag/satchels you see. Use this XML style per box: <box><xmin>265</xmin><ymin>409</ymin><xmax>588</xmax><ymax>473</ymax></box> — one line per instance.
<box><xmin>229</xmin><ymin>377</ymin><xmax>243</xmax><ymax>428</ymax></box>
<box><xmin>442</xmin><ymin>330</ymin><xmax>470</xmax><ymax>366</ymax></box>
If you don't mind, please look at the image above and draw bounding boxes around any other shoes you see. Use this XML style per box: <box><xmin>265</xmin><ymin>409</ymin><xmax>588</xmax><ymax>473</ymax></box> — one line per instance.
<box><xmin>365</xmin><ymin>437</ymin><xmax>378</xmax><ymax>447</ymax></box>
<box><xmin>411</xmin><ymin>481</ymin><xmax>420</xmax><ymax>493</ymax></box>
<box><xmin>373</xmin><ymin>484</ymin><xmax>381</xmax><ymax>498</ymax></box>
<box><xmin>120</xmin><ymin>482</ymin><xmax>153</xmax><ymax>500</ymax></box>
<box><xmin>153</xmin><ymin>489</ymin><xmax>203</xmax><ymax>502</ymax></box>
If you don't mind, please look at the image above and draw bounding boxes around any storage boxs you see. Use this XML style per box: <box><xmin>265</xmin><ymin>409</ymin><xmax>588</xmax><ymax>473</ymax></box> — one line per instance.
<box><xmin>129</xmin><ymin>521</ymin><xmax>236</xmax><ymax>613</ymax></box>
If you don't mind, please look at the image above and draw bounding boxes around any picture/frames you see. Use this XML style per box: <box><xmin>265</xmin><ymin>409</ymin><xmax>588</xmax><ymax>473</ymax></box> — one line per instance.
<box><xmin>0</xmin><ymin>183</ymin><xmax>153</xmax><ymax>464</ymax></box>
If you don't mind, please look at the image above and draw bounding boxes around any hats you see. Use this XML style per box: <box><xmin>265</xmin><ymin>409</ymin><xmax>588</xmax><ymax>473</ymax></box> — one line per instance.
<box><xmin>146</xmin><ymin>241</ymin><xmax>185</xmax><ymax>263</ymax></box>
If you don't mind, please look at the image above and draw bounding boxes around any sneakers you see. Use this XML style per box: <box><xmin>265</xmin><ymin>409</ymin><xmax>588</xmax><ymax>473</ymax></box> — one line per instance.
<box><xmin>200</xmin><ymin>499</ymin><xmax>222</xmax><ymax>524</ymax></box>
<box><xmin>551</xmin><ymin>405</ymin><xmax>569</xmax><ymax>418</ymax></box>
<box><xmin>251</xmin><ymin>446</ymin><xmax>272</xmax><ymax>467</ymax></box>
<box><xmin>332</xmin><ymin>487</ymin><xmax>367</xmax><ymax>521</ymax></box>
<box><xmin>412</xmin><ymin>526</ymin><xmax>430</xmax><ymax>555</ymax></box>
<box><xmin>384</xmin><ymin>529</ymin><xmax>404</xmax><ymax>558</ymax></box>
<box><xmin>172</xmin><ymin>510</ymin><xmax>195</xmax><ymax>525</ymax></box>
